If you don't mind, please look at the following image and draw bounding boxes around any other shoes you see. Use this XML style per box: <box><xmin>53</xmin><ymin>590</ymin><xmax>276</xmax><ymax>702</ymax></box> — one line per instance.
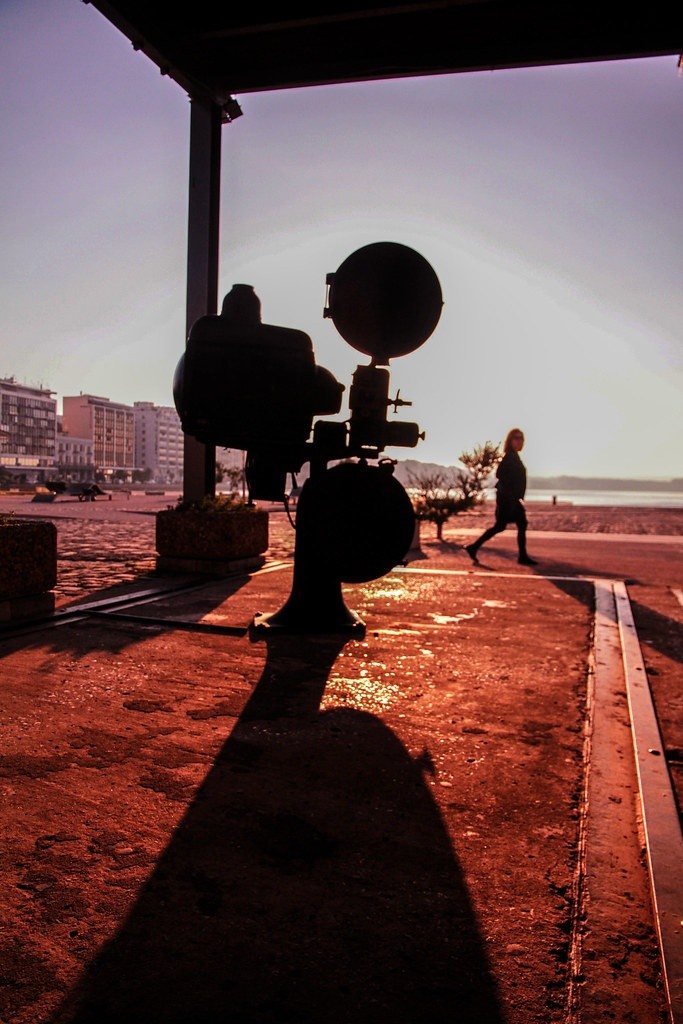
<box><xmin>464</xmin><ymin>544</ymin><xmax>479</xmax><ymax>562</ymax></box>
<box><xmin>518</xmin><ymin>556</ymin><xmax>538</xmax><ymax>565</ymax></box>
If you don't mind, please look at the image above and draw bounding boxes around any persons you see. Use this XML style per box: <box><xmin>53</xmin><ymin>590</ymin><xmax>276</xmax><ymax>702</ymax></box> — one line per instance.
<box><xmin>468</xmin><ymin>429</ymin><xmax>538</xmax><ymax>565</ymax></box>
